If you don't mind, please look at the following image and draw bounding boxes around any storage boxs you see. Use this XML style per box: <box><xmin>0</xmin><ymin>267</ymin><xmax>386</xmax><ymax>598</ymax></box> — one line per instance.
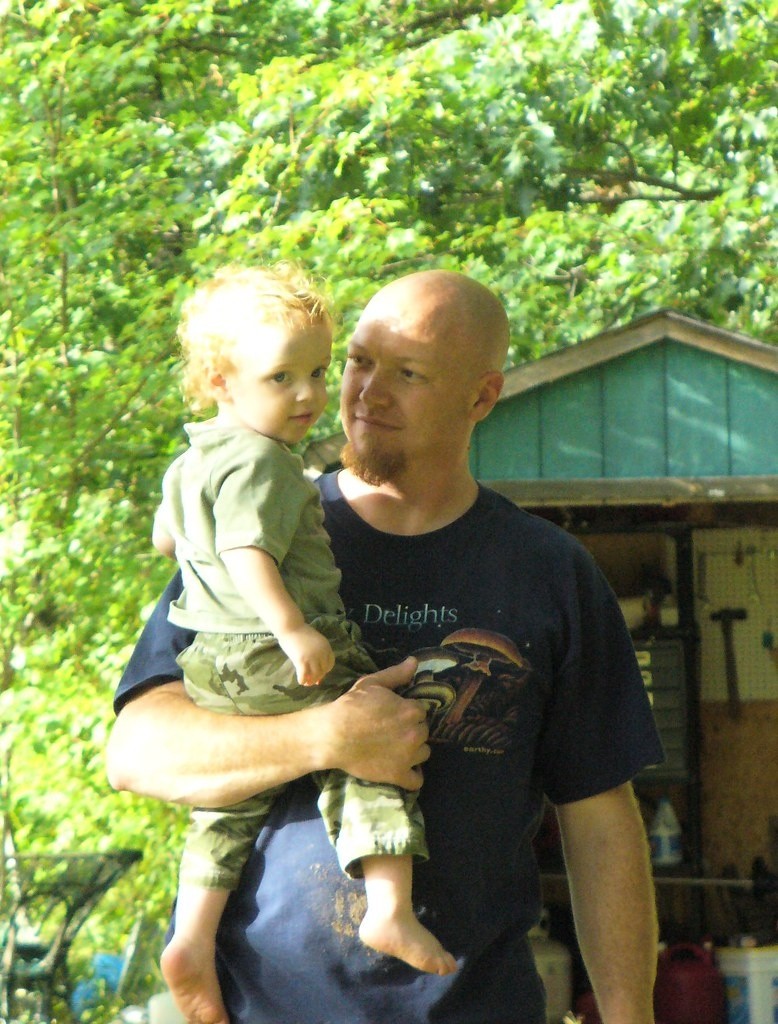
<box><xmin>714</xmin><ymin>946</ymin><xmax>778</xmax><ymax>1024</ymax></box>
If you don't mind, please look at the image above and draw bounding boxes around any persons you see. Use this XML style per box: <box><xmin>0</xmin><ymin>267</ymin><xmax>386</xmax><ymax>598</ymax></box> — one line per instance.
<box><xmin>137</xmin><ymin>260</ymin><xmax>451</xmax><ymax>1024</ymax></box>
<box><xmin>105</xmin><ymin>274</ymin><xmax>663</xmax><ymax>1024</ymax></box>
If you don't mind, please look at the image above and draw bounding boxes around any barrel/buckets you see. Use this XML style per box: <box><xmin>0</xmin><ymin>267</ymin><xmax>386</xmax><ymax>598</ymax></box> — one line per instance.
<box><xmin>714</xmin><ymin>946</ymin><xmax>778</xmax><ymax>1024</ymax></box>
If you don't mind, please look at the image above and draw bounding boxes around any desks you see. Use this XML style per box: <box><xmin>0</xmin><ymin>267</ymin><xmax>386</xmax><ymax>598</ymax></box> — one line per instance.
<box><xmin>544</xmin><ymin>874</ymin><xmax>762</xmax><ymax>943</ymax></box>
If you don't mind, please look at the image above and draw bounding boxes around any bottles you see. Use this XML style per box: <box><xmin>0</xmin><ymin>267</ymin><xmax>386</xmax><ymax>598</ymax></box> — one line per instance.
<box><xmin>648</xmin><ymin>794</ymin><xmax>686</xmax><ymax>894</ymax></box>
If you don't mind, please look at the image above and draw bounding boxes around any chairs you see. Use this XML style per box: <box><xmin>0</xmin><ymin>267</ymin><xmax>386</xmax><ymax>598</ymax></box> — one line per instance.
<box><xmin>1</xmin><ymin>850</ymin><xmax>144</xmax><ymax>1023</ymax></box>
